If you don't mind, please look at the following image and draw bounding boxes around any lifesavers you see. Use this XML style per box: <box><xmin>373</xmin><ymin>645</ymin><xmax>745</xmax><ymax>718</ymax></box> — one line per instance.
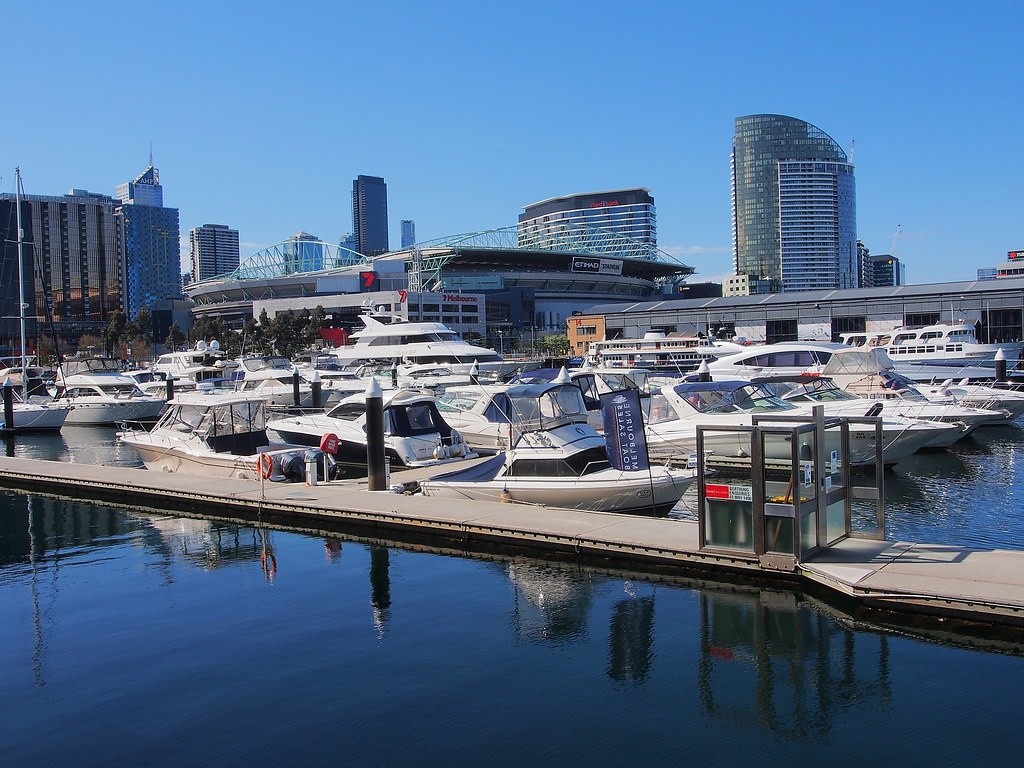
<box><xmin>256</xmin><ymin>452</ymin><xmax>273</xmax><ymax>480</ymax></box>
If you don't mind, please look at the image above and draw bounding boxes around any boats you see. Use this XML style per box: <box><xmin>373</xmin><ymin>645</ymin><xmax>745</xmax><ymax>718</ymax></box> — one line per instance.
<box><xmin>0</xmin><ymin>165</ymin><xmax>715</xmax><ymax>517</ymax></box>
<box><xmin>632</xmin><ymin>324</ymin><xmax>1024</xmax><ymax>467</ymax></box>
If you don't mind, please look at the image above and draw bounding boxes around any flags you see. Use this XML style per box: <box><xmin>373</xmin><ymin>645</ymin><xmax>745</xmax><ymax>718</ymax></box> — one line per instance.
<box><xmin>600</xmin><ymin>390</ymin><xmax>650</xmax><ymax>472</ymax></box>
